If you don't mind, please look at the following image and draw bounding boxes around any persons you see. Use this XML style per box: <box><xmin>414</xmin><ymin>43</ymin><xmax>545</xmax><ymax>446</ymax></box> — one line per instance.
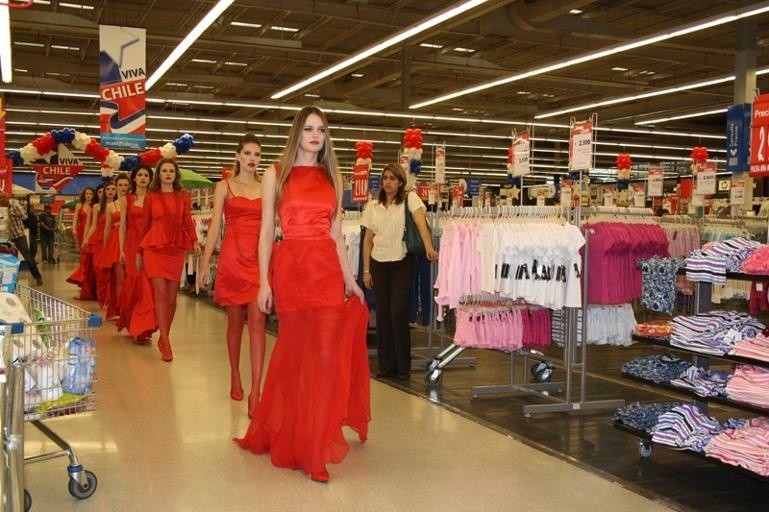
<box><xmin>199</xmin><ymin>133</ymin><xmax>266</xmax><ymax>419</ymax></box>
<box><xmin>361</xmin><ymin>162</ymin><xmax>439</xmax><ymax>382</ymax></box>
<box><xmin>233</xmin><ymin>106</ymin><xmax>371</xmax><ymax>482</ymax></box>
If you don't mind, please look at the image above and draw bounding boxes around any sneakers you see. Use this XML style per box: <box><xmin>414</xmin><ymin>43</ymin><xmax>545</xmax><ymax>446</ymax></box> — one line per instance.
<box><xmin>375</xmin><ymin>366</ymin><xmax>412</xmax><ymax>381</ymax></box>
<box><xmin>31</xmin><ymin>255</ymin><xmax>57</xmax><ymax>287</ymax></box>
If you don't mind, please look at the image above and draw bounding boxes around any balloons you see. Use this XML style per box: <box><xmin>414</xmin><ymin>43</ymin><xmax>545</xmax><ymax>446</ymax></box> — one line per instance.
<box><xmin>506</xmin><ymin>148</ymin><xmax>521</xmax><ymax>189</ymax></box>
<box><xmin>571</xmin><ymin>170</ymin><xmax>589</xmax><ymax>180</ymax></box>
<box><xmin>403</xmin><ymin>128</ymin><xmax>423</xmax><ymax>174</ymax></box>
<box><xmin>5</xmin><ymin>127</ymin><xmax>194</xmax><ymax>180</ymax></box>
<box><xmin>355</xmin><ymin>141</ymin><xmax>374</xmax><ymax>172</ymax></box>
<box><xmin>689</xmin><ymin>147</ymin><xmax>709</xmax><ymax>178</ymax></box>
<box><xmin>616</xmin><ymin>153</ymin><xmax>632</xmax><ymax>193</ymax></box>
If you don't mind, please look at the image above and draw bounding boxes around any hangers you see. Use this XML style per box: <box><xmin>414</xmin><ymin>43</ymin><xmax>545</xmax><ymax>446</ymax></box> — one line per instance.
<box><xmin>426</xmin><ymin>206</ymin><xmax>570</xmax><ymax>233</ymax></box>
<box><xmin>583</xmin><ymin>205</ymin><xmax>655</xmax><ymax>224</ymax></box>
<box><xmin>340</xmin><ymin>210</ymin><xmax>360</xmax><ymax>225</ymax></box>
<box><xmin>710</xmin><ymin>199</ymin><xmax>730</xmax><ymax>209</ymax></box>
<box><xmin>192</xmin><ymin>207</ymin><xmax>224</xmax><ymax>222</ymax></box>
<box><xmin>455</xmin><ymin>292</ymin><xmax>633</xmax><ymax>322</ymax></box>
<box><xmin>658</xmin><ymin>215</ymin><xmax>695</xmax><ymax>228</ymax></box>
<box><xmin>703</xmin><ymin>219</ymin><xmax>767</xmax><ymax>236</ymax></box>
<box><xmin>754</xmin><ymin>198</ymin><xmax>769</xmax><ymax>208</ymax></box>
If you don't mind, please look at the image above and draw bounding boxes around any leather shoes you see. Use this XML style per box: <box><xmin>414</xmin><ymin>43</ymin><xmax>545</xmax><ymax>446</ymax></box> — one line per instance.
<box><xmin>310</xmin><ymin>466</ymin><xmax>330</xmax><ymax>483</ymax></box>
<box><xmin>230</xmin><ymin>387</ymin><xmax>244</xmax><ymax>401</ymax></box>
<box><xmin>156</xmin><ymin>335</ymin><xmax>174</xmax><ymax>363</ymax></box>
<box><xmin>247</xmin><ymin>393</ymin><xmax>260</xmax><ymax>419</ymax></box>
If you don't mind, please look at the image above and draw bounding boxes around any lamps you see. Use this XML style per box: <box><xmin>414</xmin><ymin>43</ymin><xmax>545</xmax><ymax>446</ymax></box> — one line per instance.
<box><xmin>410</xmin><ymin>4</ymin><xmax>769</xmax><ymax>110</ymax></box>
<box><xmin>420</xmin><ymin>43</ymin><xmax>475</xmax><ymax>54</ymax></box>
<box><xmin>533</xmin><ymin>66</ymin><xmax>768</xmax><ymax>120</ymax></box>
<box><xmin>145</xmin><ymin>0</ymin><xmax>233</xmax><ymax>92</ymax></box>
<box><xmin>230</xmin><ymin>20</ymin><xmax>300</xmax><ymax>33</ymax></box>
<box><xmin>634</xmin><ymin>106</ymin><xmax>729</xmax><ymax>126</ymax></box>
<box><xmin>165</xmin><ymin>83</ymin><xmax>213</xmax><ymax>90</ymax></box>
<box><xmin>191</xmin><ymin>58</ymin><xmax>248</xmax><ymax>68</ymax></box>
<box><xmin>270</xmin><ymin>0</ymin><xmax>487</xmax><ymax>101</ymax></box>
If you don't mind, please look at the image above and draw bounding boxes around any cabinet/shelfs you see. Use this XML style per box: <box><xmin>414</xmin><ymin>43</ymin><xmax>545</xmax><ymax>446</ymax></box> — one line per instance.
<box><xmin>614</xmin><ymin>260</ymin><xmax>769</xmax><ymax>483</ymax></box>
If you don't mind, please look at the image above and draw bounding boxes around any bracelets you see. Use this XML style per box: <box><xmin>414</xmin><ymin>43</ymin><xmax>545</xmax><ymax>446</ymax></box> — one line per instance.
<box><xmin>363</xmin><ymin>270</ymin><xmax>370</xmax><ymax>274</ymax></box>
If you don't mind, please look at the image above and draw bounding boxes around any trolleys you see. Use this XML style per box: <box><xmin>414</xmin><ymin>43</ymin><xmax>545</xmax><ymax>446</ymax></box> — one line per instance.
<box><xmin>55</xmin><ymin>226</ymin><xmax>76</xmax><ymax>264</ymax></box>
<box><xmin>0</xmin><ymin>242</ymin><xmax>102</xmax><ymax>512</ymax></box>
<box><xmin>411</xmin><ymin>236</ymin><xmax>570</xmax><ymax>400</ymax></box>
